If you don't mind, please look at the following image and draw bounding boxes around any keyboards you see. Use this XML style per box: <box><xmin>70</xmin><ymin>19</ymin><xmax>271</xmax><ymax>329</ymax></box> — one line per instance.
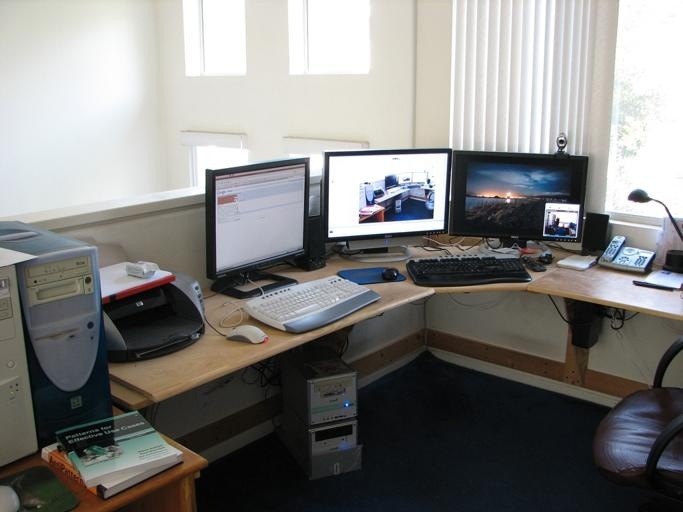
<box><xmin>406</xmin><ymin>258</ymin><xmax>531</xmax><ymax>287</ymax></box>
<box><xmin>245</xmin><ymin>276</ymin><xmax>383</xmax><ymax>335</ymax></box>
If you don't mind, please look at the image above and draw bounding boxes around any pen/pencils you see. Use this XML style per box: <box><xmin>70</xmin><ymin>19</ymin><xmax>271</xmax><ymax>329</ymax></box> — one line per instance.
<box><xmin>633</xmin><ymin>280</ymin><xmax>674</xmax><ymax>291</ymax></box>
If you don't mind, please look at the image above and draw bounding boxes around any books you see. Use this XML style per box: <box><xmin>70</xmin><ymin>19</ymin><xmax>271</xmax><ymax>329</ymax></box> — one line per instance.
<box><xmin>557</xmin><ymin>254</ymin><xmax>598</xmax><ymax>270</ymax></box>
<box><xmin>41</xmin><ymin>409</ymin><xmax>183</xmax><ymax>501</ymax></box>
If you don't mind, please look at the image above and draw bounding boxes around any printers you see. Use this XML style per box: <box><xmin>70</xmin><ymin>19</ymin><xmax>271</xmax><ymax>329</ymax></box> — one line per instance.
<box><xmin>97</xmin><ymin>262</ymin><xmax>205</xmax><ymax>363</ymax></box>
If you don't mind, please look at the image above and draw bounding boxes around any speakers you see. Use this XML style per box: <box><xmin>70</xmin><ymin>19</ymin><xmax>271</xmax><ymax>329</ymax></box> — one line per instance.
<box><xmin>582</xmin><ymin>212</ymin><xmax>608</xmax><ymax>251</ymax></box>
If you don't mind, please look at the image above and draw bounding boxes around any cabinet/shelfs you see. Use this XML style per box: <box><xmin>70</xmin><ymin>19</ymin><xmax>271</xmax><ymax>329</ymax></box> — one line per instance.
<box><xmin>0</xmin><ymin>404</ymin><xmax>207</xmax><ymax>512</ymax></box>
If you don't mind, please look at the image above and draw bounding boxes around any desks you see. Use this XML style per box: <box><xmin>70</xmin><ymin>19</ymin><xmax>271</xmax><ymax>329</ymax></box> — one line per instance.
<box><xmin>108</xmin><ymin>233</ymin><xmax>681</xmax><ymax>412</ymax></box>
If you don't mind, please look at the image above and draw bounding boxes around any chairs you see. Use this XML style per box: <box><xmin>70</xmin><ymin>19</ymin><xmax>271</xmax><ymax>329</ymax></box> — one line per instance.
<box><xmin>588</xmin><ymin>337</ymin><xmax>682</xmax><ymax>511</ymax></box>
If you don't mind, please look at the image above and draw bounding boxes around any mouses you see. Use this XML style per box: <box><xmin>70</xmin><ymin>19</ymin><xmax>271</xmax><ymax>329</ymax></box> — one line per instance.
<box><xmin>383</xmin><ymin>269</ymin><xmax>399</xmax><ymax>282</ymax></box>
<box><xmin>227</xmin><ymin>325</ymin><xmax>268</xmax><ymax>346</ymax></box>
<box><xmin>538</xmin><ymin>250</ymin><xmax>552</xmax><ymax>265</ymax></box>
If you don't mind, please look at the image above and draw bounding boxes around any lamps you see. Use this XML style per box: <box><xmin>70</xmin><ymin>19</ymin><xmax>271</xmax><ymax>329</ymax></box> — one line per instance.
<box><xmin>627</xmin><ymin>189</ymin><xmax>682</xmax><ymax>240</ymax></box>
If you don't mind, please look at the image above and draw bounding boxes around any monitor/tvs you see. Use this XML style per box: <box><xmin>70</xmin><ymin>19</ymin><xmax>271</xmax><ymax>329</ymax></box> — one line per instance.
<box><xmin>321</xmin><ymin>150</ymin><xmax>453</xmax><ymax>264</ymax></box>
<box><xmin>207</xmin><ymin>158</ymin><xmax>308</xmax><ymax>298</ymax></box>
<box><xmin>450</xmin><ymin>151</ymin><xmax>587</xmax><ymax>250</ymax></box>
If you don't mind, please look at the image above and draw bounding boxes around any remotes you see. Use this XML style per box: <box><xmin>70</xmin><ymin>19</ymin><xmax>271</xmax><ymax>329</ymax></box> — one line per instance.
<box><xmin>522</xmin><ymin>256</ymin><xmax>548</xmax><ymax>275</ymax></box>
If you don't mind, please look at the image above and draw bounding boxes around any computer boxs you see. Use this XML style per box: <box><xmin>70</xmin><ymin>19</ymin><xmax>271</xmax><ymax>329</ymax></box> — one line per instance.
<box><xmin>0</xmin><ymin>221</ymin><xmax>114</xmax><ymax>455</ymax></box>
<box><xmin>284</xmin><ymin>353</ymin><xmax>362</xmax><ymax>481</ymax></box>
<box><xmin>0</xmin><ymin>265</ymin><xmax>38</xmax><ymax>468</ymax></box>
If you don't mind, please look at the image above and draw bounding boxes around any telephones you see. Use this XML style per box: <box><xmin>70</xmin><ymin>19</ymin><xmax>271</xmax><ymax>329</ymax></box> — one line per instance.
<box><xmin>598</xmin><ymin>235</ymin><xmax>656</xmax><ymax>274</ymax></box>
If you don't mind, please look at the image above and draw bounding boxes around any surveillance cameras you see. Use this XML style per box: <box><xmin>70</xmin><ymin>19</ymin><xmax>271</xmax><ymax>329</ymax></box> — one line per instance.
<box><xmin>557</xmin><ymin>134</ymin><xmax>568</xmax><ymax>157</ymax></box>
